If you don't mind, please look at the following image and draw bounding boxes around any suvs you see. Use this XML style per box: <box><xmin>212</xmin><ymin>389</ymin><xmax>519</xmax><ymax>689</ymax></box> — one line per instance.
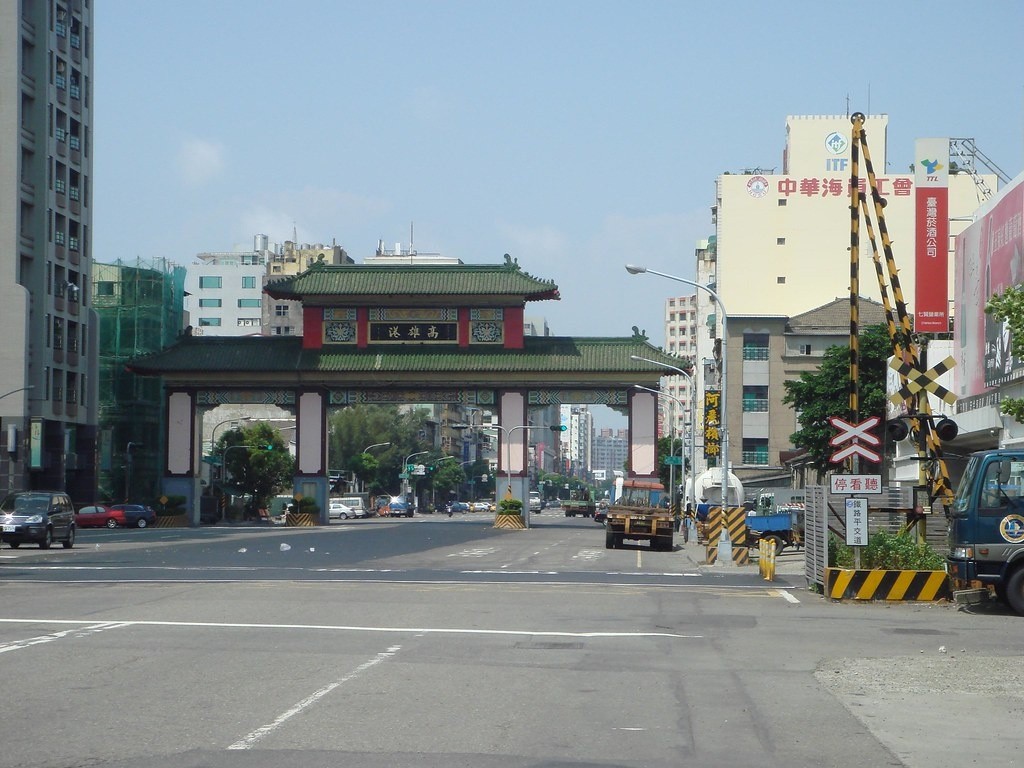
<box><xmin>0</xmin><ymin>490</ymin><xmax>76</xmax><ymax>550</ymax></box>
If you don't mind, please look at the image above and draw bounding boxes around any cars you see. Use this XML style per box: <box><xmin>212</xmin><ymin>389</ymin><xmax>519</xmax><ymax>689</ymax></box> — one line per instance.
<box><xmin>329</xmin><ymin>504</ymin><xmax>355</xmax><ymax>520</ymax></box>
<box><xmin>110</xmin><ymin>504</ymin><xmax>157</xmax><ymax>528</ymax></box>
<box><xmin>75</xmin><ymin>503</ymin><xmax>127</xmax><ymax>529</ymax></box>
<box><xmin>595</xmin><ymin>508</ymin><xmax>610</xmax><ymax>527</ymax></box>
<box><xmin>450</xmin><ymin>500</ymin><xmax>496</xmax><ymax>514</ymax></box>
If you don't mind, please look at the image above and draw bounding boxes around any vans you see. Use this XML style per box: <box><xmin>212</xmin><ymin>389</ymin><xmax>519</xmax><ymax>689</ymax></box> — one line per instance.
<box><xmin>330</xmin><ymin>497</ymin><xmax>366</xmax><ymax>518</ymax></box>
<box><xmin>529</xmin><ymin>491</ymin><xmax>541</xmax><ymax>514</ymax></box>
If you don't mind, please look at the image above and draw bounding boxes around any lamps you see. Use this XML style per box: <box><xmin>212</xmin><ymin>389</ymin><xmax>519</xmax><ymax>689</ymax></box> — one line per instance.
<box><xmin>63</xmin><ymin>279</ymin><xmax>80</xmax><ymax>291</ymax></box>
<box><xmin>7</xmin><ymin>423</ymin><xmax>17</xmax><ymax>452</ymax></box>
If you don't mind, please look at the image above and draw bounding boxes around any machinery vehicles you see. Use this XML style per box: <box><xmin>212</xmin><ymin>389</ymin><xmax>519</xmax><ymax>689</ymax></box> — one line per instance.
<box><xmin>679</xmin><ymin>466</ymin><xmax>745</xmax><ymax>518</ymax></box>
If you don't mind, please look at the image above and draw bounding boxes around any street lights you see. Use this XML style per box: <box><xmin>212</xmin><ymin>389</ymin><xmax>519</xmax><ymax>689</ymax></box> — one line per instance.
<box><xmin>362</xmin><ymin>442</ymin><xmax>390</xmax><ymax>493</ymax></box>
<box><xmin>629</xmin><ymin>355</ymin><xmax>698</xmax><ymax>543</ymax></box>
<box><xmin>406</xmin><ymin>451</ymin><xmax>429</xmax><ymax>497</ymax></box>
<box><xmin>433</xmin><ymin>456</ymin><xmax>454</xmax><ymax>501</ymax></box>
<box><xmin>210</xmin><ymin>415</ymin><xmax>252</xmax><ymax>495</ymax></box>
<box><xmin>456</xmin><ymin>459</ymin><xmax>476</xmax><ymax>502</ymax></box>
<box><xmin>634</xmin><ymin>385</ymin><xmax>687</xmax><ymax>537</ymax></box>
<box><xmin>626</xmin><ymin>263</ymin><xmax>736</xmax><ymax>569</ymax></box>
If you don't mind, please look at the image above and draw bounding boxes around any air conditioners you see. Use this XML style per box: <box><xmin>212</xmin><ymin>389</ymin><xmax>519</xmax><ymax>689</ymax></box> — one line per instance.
<box><xmin>245</xmin><ymin>320</ymin><xmax>252</xmax><ymax>326</ymax></box>
<box><xmin>238</xmin><ymin>320</ymin><xmax>244</xmax><ymax>326</ymax></box>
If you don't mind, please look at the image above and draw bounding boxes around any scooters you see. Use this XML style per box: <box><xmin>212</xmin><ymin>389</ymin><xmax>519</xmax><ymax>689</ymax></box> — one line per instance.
<box><xmin>445</xmin><ymin>504</ymin><xmax>454</xmax><ymax>517</ymax></box>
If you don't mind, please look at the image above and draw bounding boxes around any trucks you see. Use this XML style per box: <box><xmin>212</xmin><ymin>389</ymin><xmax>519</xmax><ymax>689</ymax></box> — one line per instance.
<box><xmin>605</xmin><ymin>480</ymin><xmax>675</xmax><ymax>551</ymax></box>
<box><xmin>927</xmin><ymin>449</ymin><xmax>1024</xmax><ymax>617</ymax></box>
<box><xmin>561</xmin><ymin>490</ymin><xmax>596</xmax><ymax>518</ymax></box>
<box><xmin>745</xmin><ymin>509</ymin><xmax>805</xmax><ymax>555</ymax></box>
<box><xmin>388</xmin><ymin>495</ymin><xmax>416</xmax><ymax>517</ymax></box>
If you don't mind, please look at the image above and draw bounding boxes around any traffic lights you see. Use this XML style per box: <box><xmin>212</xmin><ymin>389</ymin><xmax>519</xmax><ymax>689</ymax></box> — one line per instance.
<box><xmin>429</xmin><ymin>464</ymin><xmax>433</xmax><ymax>474</ymax></box>
<box><xmin>258</xmin><ymin>445</ymin><xmax>273</xmax><ymax>450</ymax></box>
<box><xmin>550</xmin><ymin>425</ymin><xmax>568</xmax><ymax>431</ymax></box>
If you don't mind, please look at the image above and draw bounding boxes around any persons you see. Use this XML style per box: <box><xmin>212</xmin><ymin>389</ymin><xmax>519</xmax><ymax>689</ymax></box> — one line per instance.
<box><xmin>445</xmin><ymin>500</ymin><xmax>454</xmax><ymax>515</ymax></box>
